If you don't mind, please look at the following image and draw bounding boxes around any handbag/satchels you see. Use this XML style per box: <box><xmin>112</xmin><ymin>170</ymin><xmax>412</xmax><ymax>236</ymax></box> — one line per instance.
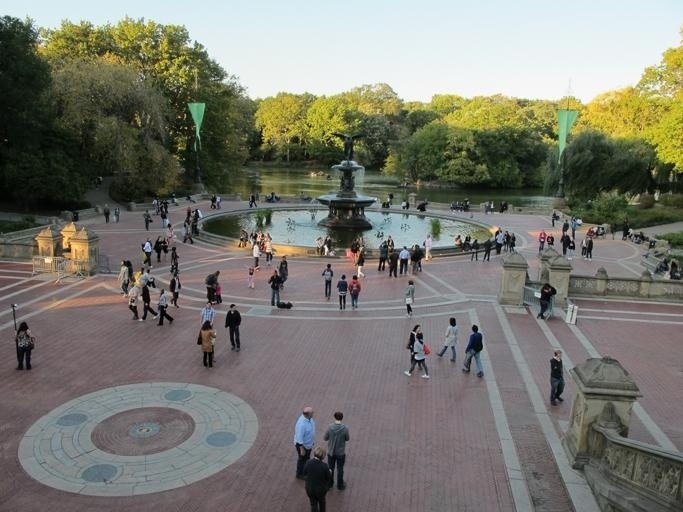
<box><xmin>158</xmin><ymin>304</ymin><xmax>165</xmax><ymax>311</ymax></box>
<box><xmin>405</xmin><ymin>297</ymin><xmax>413</xmax><ymax>305</ymax></box>
<box><xmin>130</xmin><ymin>298</ymin><xmax>135</xmax><ymax>304</ymax></box>
<box><xmin>197</xmin><ymin>334</ymin><xmax>202</xmax><ymax>346</ymax></box>
<box><xmin>184</xmin><ymin>234</ymin><xmax>192</xmax><ymax>240</ymax></box>
<box><xmin>17</xmin><ymin>330</ymin><xmax>30</xmax><ymax>348</ymax></box>
<box><xmin>423</xmin><ymin>343</ymin><xmax>432</xmax><ymax>355</ymax></box>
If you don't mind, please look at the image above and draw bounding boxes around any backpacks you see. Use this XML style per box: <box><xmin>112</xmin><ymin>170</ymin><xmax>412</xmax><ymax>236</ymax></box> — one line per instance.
<box><xmin>352</xmin><ymin>283</ymin><xmax>359</xmax><ymax>296</ymax></box>
<box><xmin>324</xmin><ymin>271</ymin><xmax>332</xmax><ymax>281</ymax></box>
<box><xmin>141</xmin><ymin>243</ymin><xmax>146</xmax><ymax>252</ymax></box>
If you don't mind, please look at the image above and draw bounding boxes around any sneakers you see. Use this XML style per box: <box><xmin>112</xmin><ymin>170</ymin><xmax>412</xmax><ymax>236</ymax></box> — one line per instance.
<box><xmin>142</xmin><ymin>261</ymin><xmax>153</xmax><ymax>269</ymax></box>
<box><xmin>169</xmin><ymin>319</ymin><xmax>174</xmax><ymax>324</ymax></box>
<box><xmin>462</xmin><ymin>368</ymin><xmax>470</xmax><ymax>372</ymax></box>
<box><xmin>551</xmin><ymin>400</ymin><xmax>557</xmax><ymax>405</ymax></box>
<box><xmin>403</xmin><ymin>370</ymin><xmax>412</xmax><ymax>376</ymax></box>
<box><xmin>421</xmin><ymin>374</ymin><xmax>429</xmax><ymax>379</ymax></box>
<box><xmin>152</xmin><ymin>313</ymin><xmax>160</xmax><ymax>320</ymax></box>
<box><xmin>138</xmin><ymin>319</ymin><xmax>146</xmax><ymax>322</ymax></box>
<box><xmin>157</xmin><ymin>324</ymin><xmax>163</xmax><ymax>326</ymax></box>
<box><xmin>231</xmin><ymin>346</ymin><xmax>241</xmax><ymax>353</ymax></box>
<box><xmin>584</xmin><ymin>257</ymin><xmax>592</xmax><ymax>262</ymax></box>
<box><xmin>450</xmin><ymin>358</ymin><xmax>456</xmax><ymax>362</ymax></box>
<box><xmin>557</xmin><ymin>397</ymin><xmax>564</xmax><ymax>402</ymax></box>
<box><xmin>338</xmin><ymin>482</ymin><xmax>346</xmax><ymax>489</ymax></box>
<box><xmin>477</xmin><ymin>373</ymin><xmax>483</xmax><ymax>378</ymax></box>
<box><xmin>435</xmin><ymin>352</ymin><xmax>442</xmax><ymax>357</ymax></box>
<box><xmin>15</xmin><ymin>366</ymin><xmax>33</xmax><ymax>370</ymax></box>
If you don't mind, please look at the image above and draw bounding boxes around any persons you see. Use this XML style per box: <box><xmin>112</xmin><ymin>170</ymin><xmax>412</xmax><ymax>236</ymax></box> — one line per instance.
<box><xmin>72</xmin><ymin>192</ymin><xmax>290</xmax><ymax>367</ymax></box>
<box><xmin>15</xmin><ymin>322</ymin><xmax>34</xmax><ymax>370</ymax></box>
<box><xmin>455</xmin><ymin>226</ymin><xmax>516</xmax><ymax>262</ymax></box>
<box><xmin>403</xmin><ymin>281</ymin><xmax>415</xmax><ymax>316</ymax></box>
<box><xmin>337</xmin><ymin>275</ymin><xmax>348</xmax><ymax>309</ymax></box>
<box><xmin>550</xmin><ymin>350</ymin><xmax>566</xmax><ymax>405</ymax></box>
<box><xmin>322</xmin><ymin>264</ymin><xmax>333</xmax><ymax>300</ymax></box>
<box><xmin>294</xmin><ymin>406</ymin><xmax>349</xmax><ymax>512</ymax></box>
<box><xmin>348</xmin><ymin>275</ymin><xmax>361</xmax><ymax>308</ymax></box>
<box><xmin>315</xmin><ymin>234</ymin><xmax>433</xmax><ymax>277</ymax></box>
<box><xmin>537</xmin><ymin>283</ymin><xmax>556</xmax><ymax>319</ymax></box>
<box><xmin>539</xmin><ymin>210</ymin><xmax>680</xmax><ymax>279</ymax></box>
<box><xmin>403</xmin><ymin>318</ymin><xmax>484</xmax><ymax>378</ymax></box>
<box><xmin>382</xmin><ymin>192</ymin><xmax>507</xmax><ymax>215</ymax></box>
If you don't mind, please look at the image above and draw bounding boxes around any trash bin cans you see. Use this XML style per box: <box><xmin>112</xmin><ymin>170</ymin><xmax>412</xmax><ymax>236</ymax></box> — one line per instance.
<box><xmin>259</xmin><ymin>194</ymin><xmax>265</xmax><ymax>202</ymax></box>
<box><xmin>72</xmin><ymin>212</ymin><xmax>78</xmax><ymax>221</ymax></box>
